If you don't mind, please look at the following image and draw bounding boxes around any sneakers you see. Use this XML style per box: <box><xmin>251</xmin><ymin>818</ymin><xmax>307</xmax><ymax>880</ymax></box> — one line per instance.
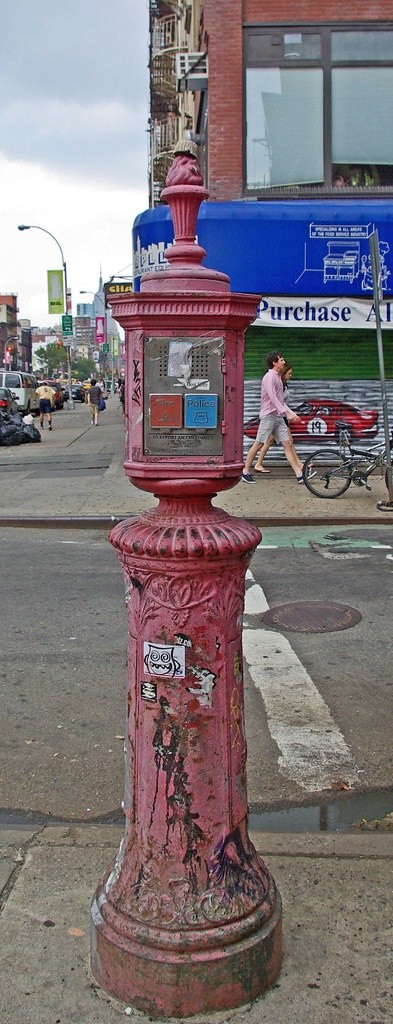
<box><xmin>296</xmin><ymin>469</ymin><xmax>318</xmax><ymax>485</ymax></box>
<box><xmin>241</xmin><ymin>472</ymin><xmax>257</xmax><ymax>484</ymax></box>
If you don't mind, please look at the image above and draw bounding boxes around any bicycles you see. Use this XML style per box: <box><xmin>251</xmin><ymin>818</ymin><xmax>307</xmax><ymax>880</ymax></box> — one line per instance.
<box><xmin>301</xmin><ymin>419</ymin><xmax>393</xmax><ymax>497</ymax></box>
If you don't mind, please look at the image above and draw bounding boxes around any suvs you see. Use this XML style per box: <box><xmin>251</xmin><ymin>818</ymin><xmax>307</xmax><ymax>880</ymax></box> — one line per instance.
<box><xmin>39</xmin><ymin>379</ymin><xmax>65</xmax><ymax>411</ymax></box>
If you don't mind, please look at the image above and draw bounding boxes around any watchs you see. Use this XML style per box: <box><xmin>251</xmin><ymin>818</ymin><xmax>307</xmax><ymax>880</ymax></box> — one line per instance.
<box><xmin>33</xmin><ymin>399</ymin><xmax>37</xmax><ymax>401</ymax></box>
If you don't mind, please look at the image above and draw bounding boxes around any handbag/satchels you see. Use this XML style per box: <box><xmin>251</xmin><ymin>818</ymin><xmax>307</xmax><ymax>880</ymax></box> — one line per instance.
<box><xmin>98</xmin><ymin>399</ymin><xmax>106</xmax><ymax>412</ymax></box>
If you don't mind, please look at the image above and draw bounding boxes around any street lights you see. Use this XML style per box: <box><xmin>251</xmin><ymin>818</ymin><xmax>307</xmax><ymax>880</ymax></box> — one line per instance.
<box><xmin>79</xmin><ymin>290</ymin><xmax>110</xmax><ymax>398</ymax></box>
<box><xmin>3</xmin><ymin>336</ymin><xmax>18</xmax><ymax>370</ymax></box>
<box><xmin>17</xmin><ymin>223</ymin><xmax>75</xmax><ymax>412</ymax></box>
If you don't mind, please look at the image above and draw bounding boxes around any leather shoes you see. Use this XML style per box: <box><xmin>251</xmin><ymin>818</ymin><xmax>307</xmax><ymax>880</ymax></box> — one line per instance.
<box><xmin>254</xmin><ymin>465</ymin><xmax>271</xmax><ymax>473</ymax></box>
<box><xmin>299</xmin><ymin>463</ymin><xmax>314</xmax><ymax>469</ymax></box>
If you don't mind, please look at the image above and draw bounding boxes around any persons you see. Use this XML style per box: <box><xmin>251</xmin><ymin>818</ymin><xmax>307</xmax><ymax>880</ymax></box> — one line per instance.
<box><xmin>95</xmin><ymin>379</ymin><xmax>104</xmax><ymax>397</ymax></box>
<box><xmin>252</xmin><ymin>362</ymin><xmax>314</xmax><ymax>474</ymax></box>
<box><xmin>33</xmin><ymin>382</ymin><xmax>56</xmax><ymax>431</ymax></box>
<box><xmin>85</xmin><ymin>379</ymin><xmax>102</xmax><ymax>426</ymax></box>
<box><xmin>120</xmin><ymin>379</ymin><xmax>126</xmax><ymax>422</ymax></box>
<box><xmin>241</xmin><ymin>351</ymin><xmax>318</xmax><ymax>486</ymax></box>
<box><xmin>101</xmin><ymin>380</ymin><xmax>105</xmax><ymax>390</ymax></box>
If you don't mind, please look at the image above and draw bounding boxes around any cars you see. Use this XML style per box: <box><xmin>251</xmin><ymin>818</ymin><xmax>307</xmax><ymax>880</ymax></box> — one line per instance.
<box><xmin>63</xmin><ymin>383</ymin><xmax>87</xmax><ymax>402</ymax></box>
<box><xmin>0</xmin><ymin>388</ymin><xmax>20</xmax><ymax>416</ymax></box>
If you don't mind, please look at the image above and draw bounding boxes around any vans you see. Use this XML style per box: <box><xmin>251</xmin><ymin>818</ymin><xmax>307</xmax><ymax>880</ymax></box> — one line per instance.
<box><xmin>0</xmin><ymin>370</ymin><xmax>42</xmax><ymax>417</ymax></box>
